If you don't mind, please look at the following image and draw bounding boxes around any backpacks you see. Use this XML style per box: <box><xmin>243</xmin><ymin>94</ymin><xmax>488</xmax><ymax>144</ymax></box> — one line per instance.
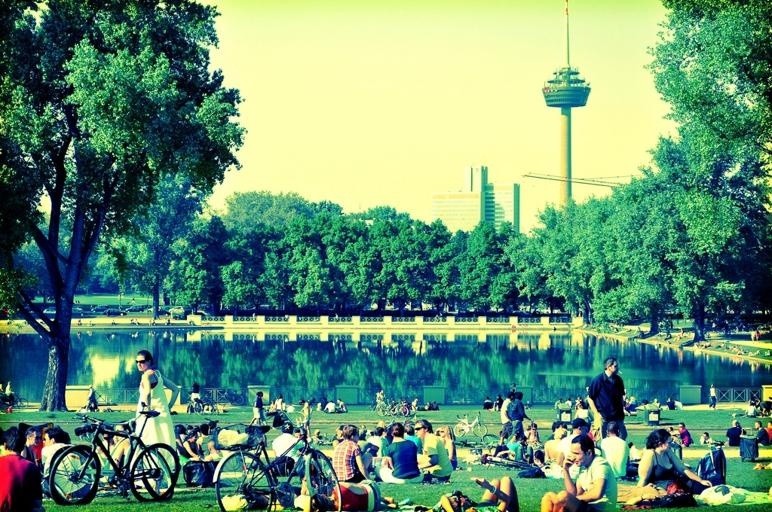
<box><xmin>697</xmin><ymin>450</ymin><xmax>726</xmax><ymax>485</ymax></box>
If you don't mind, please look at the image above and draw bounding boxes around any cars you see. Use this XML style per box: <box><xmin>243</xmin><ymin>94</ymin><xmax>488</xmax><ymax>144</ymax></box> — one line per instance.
<box><xmin>43</xmin><ymin>305</ymin><xmax>210</xmax><ymax>320</ymax></box>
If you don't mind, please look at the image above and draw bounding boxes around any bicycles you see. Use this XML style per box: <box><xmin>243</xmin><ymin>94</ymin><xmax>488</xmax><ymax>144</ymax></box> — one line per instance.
<box><xmin>48</xmin><ymin>410</ymin><xmax>181</xmax><ymax>506</ymax></box>
<box><xmin>224</xmin><ymin>391</ymin><xmax>245</xmax><ymax>406</ymax></box>
<box><xmin>0</xmin><ymin>393</ymin><xmax>28</xmax><ymax>409</ymax></box>
<box><xmin>213</xmin><ymin>397</ymin><xmax>343</xmax><ymax>512</ymax></box>
<box><xmin>453</xmin><ymin>412</ymin><xmax>489</xmax><ymax>438</ymax></box>
<box><xmin>374</xmin><ymin>399</ymin><xmax>417</xmax><ymax>418</ymax></box>
<box><xmin>187</xmin><ymin>400</ymin><xmax>205</xmax><ymax>415</ymax></box>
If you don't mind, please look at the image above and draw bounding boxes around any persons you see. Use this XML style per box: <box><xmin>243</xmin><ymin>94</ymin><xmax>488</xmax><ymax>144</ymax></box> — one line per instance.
<box><xmin>0</xmin><ymin>380</ymin><xmax>772</xmax><ymax>512</ymax></box>
<box><xmin>2</xmin><ymin>297</ymin><xmax>173</xmax><ymax>327</ymax></box>
<box><xmin>133</xmin><ymin>349</ymin><xmax>180</xmax><ymax>498</ymax></box>
<box><xmin>586</xmin><ymin>358</ymin><xmax>627</xmax><ymax>443</ymax></box>
<box><xmin>606</xmin><ymin>315</ymin><xmax>771</xmax><ymax>358</ymax></box>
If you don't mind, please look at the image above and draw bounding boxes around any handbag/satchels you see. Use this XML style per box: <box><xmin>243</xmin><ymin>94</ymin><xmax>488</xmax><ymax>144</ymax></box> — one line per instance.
<box><xmin>272</xmin><ymin>456</ymin><xmax>294</xmax><ymax>477</ymax></box>
<box><xmin>739</xmin><ymin>428</ymin><xmax>758</xmax><ymax>462</ymax></box>
<box><xmin>183</xmin><ymin>461</ymin><xmax>219</xmax><ymax>487</ymax></box>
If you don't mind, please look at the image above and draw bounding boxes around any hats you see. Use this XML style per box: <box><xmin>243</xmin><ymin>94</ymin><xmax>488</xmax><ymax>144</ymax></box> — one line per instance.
<box><xmin>282</xmin><ymin>422</ymin><xmax>293</xmax><ymax>434</ymax></box>
<box><xmin>573</xmin><ymin>418</ymin><xmax>591</xmax><ymax>428</ymax></box>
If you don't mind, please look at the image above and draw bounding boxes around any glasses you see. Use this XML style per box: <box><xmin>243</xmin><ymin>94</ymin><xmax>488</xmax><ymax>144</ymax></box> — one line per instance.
<box><xmin>415</xmin><ymin>427</ymin><xmax>424</xmax><ymax>430</ymax></box>
<box><xmin>136</xmin><ymin>360</ymin><xmax>146</xmax><ymax>364</ymax></box>
